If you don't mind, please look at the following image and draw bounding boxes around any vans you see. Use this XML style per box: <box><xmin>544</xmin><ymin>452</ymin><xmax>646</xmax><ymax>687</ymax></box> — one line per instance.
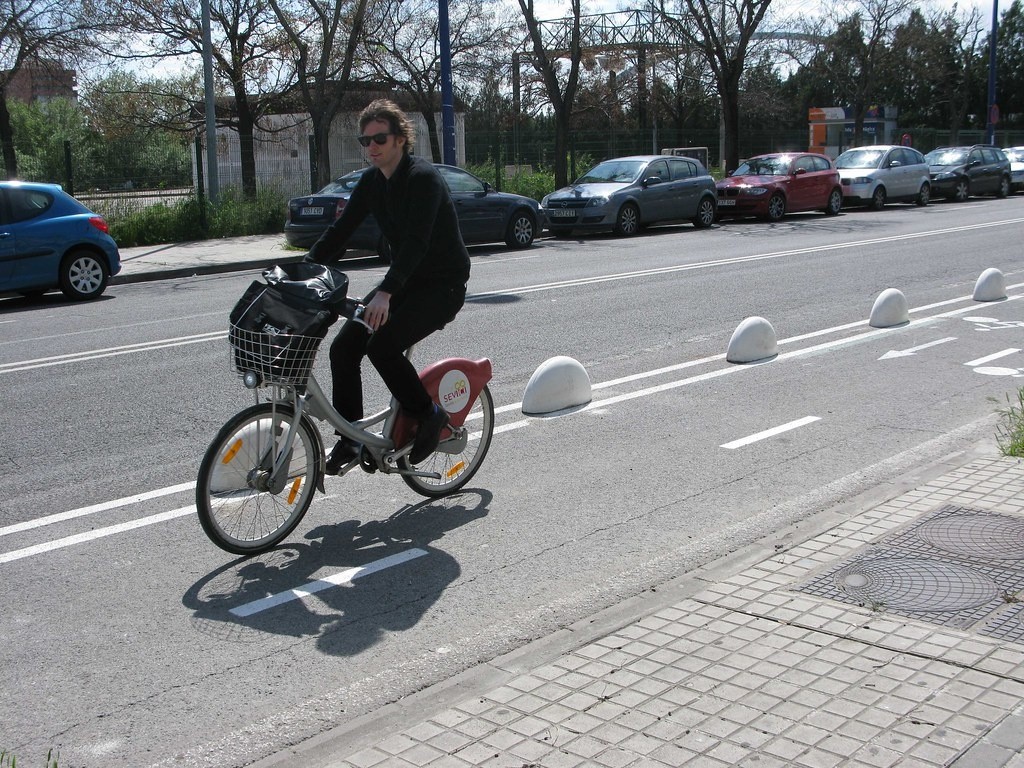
<box><xmin>544</xmin><ymin>156</ymin><xmax>722</xmax><ymax>234</ymax></box>
<box><xmin>833</xmin><ymin>145</ymin><xmax>931</xmax><ymax>210</ymax></box>
<box><xmin>1002</xmin><ymin>146</ymin><xmax>1024</xmax><ymax>194</ymax></box>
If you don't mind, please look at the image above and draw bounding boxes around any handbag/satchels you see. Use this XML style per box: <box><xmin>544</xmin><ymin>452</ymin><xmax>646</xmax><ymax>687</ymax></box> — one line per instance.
<box><xmin>229</xmin><ymin>257</ymin><xmax>350</xmax><ymax>384</ymax></box>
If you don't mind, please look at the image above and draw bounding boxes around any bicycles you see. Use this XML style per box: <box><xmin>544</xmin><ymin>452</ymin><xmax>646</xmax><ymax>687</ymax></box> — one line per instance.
<box><xmin>195</xmin><ymin>277</ymin><xmax>494</xmax><ymax>554</ymax></box>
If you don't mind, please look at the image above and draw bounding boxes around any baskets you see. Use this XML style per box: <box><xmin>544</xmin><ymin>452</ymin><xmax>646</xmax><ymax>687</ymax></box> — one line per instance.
<box><xmin>228</xmin><ymin>320</ymin><xmax>323</xmax><ymax>386</ymax></box>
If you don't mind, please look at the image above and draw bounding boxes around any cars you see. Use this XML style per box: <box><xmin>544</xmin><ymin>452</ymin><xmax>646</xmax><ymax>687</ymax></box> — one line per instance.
<box><xmin>0</xmin><ymin>181</ymin><xmax>120</xmax><ymax>299</ymax></box>
<box><xmin>715</xmin><ymin>153</ymin><xmax>843</xmax><ymax>221</ymax></box>
<box><xmin>286</xmin><ymin>163</ymin><xmax>548</xmax><ymax>249</ymax></box>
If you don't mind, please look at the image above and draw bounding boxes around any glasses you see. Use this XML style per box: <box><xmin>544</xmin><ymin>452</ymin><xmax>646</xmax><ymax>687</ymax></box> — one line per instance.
<box><xmin>357</xmin><ymin>132</ymin><xmax>392</xmax><ymax>147</ymax></box>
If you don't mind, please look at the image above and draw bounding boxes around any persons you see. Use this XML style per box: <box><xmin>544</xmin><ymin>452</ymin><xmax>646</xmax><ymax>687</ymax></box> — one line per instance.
<box><xmin>301</xmin><ymin>101</ymin><xmax>470</xmax><ymax>475</ymax></box>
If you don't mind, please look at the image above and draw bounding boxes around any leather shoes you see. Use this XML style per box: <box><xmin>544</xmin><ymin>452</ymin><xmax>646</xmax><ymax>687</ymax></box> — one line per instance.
<box><xmin>409</xmin><ymin>402</ymin><xmax>451</xmax><ymax>465</ymax></box>
<box><xmin>325</xmin><ymin>439</ymin><xmax>360</xmax><ymax>470</ymax></box>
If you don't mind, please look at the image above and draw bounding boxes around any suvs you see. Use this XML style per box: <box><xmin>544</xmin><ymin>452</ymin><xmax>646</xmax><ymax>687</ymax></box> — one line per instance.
<box><xmin>925</xmin><ymin>144</ymin><xmax>1011</xmax><ymax>201</ymax></box>
